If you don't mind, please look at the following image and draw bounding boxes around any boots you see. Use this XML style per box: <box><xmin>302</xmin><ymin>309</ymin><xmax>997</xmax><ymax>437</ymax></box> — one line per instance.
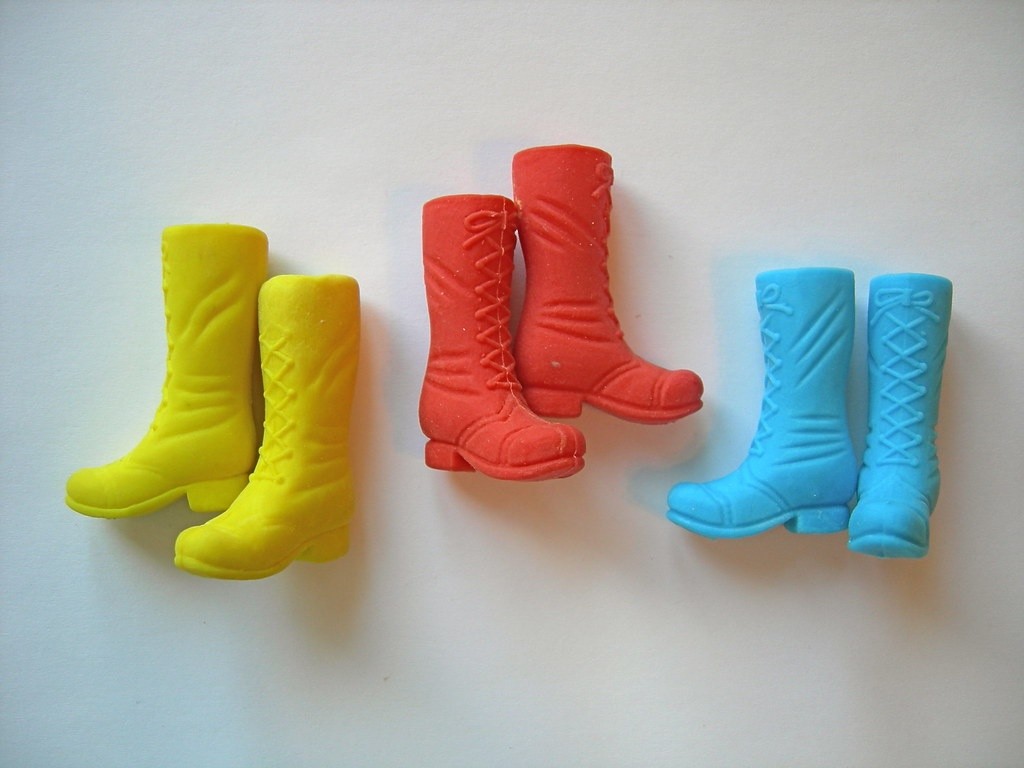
<box><xmin>418</xmin><ymin>194</ymin><xmax>587</xmax><ymax>482</ymax></box>
<box><xmin>665</xmin><ymin>268</ymin><xmax>859</xmax><ymax>540</ymax></box>
<box><xmin>512</xmin><ymin>145</ymin><xmax>703</xmax><ymax>425</ymax></box>
<box><xmin>848</xmin><ymin>272</ymin><xmax>952</xmax><ymax>556</ymax></box>
<box><xmin>64</xmin><ymin>222</ymin><xmax>269</xmax><ymax>517</ymax></box>
<box><xmin>173</xmin><ymin>273</ymin><xmax>361</xmax><ymax>578</ymax></box>
